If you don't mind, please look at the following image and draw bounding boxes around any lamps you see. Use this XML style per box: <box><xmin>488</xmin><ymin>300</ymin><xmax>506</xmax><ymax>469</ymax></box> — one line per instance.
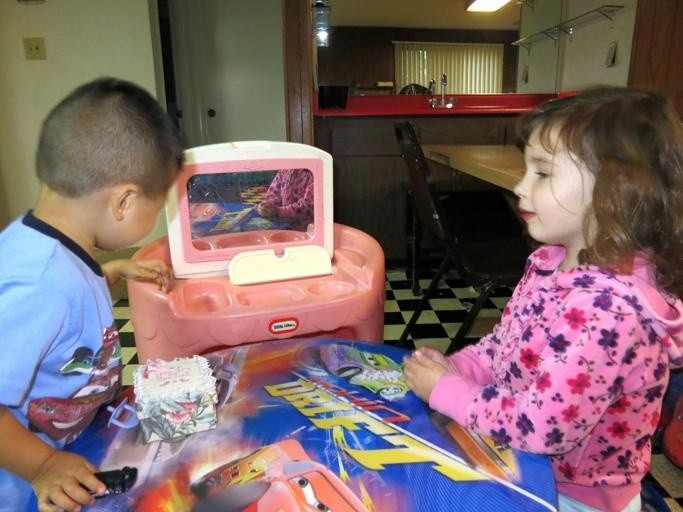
<box><xmin>466</xmin><ymin>2</ymin><xmax>513</xmax><ymax>12</ymax></box>
<box><xmin>311</xmin><ymin>0</ymin><xmax>332</xmax><ymax>46</ymax></box>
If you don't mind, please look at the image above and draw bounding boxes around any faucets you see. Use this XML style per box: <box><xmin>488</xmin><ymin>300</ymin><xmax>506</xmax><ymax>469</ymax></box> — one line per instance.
<box><xmin>428</xmin><ymin>79</ymin><xmax>435</xmax><ymax>94</ymax></box>
<box><xmin>431</xmin><ymin>73</ymin><xmax>456</xmax><ymax>108</ymax></box>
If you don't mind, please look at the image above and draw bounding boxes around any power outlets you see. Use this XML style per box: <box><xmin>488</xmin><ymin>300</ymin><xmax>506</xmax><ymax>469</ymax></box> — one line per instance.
<box><xmin>23</xmin><ymin>36</ymin><xmax>50</xmax><ymax>62</ymax></box>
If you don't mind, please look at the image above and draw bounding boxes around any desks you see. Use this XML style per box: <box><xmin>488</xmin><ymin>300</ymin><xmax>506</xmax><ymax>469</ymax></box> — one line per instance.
<box><xmin>28</xmin><ymin>336</ymin><xmax>559</xmax><ymax>512</ymax></box>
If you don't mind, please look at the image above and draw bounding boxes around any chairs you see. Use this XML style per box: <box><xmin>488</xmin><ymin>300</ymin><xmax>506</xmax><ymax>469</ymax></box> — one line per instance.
<box><xmin>392</xmin><ymin>121</ymin><xmax>539</xmax><ymax>344</ymax></box>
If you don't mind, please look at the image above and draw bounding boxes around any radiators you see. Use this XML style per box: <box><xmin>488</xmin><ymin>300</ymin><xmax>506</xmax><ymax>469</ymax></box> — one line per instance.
<box><xmin>393</xmin><ymin>41</ymin><xmax>505</xmax><ymax>96</ymax></box>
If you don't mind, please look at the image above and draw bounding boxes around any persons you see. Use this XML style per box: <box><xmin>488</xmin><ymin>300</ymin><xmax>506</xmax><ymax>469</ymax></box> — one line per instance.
<box><xmin>404</xmin><ymin>84</ymin><xmax>683</xmax><ymax>511</ymax></box>
<box><xmin>258</xmin><ymin>169</ymin><xmax>314</xmax><ymax>223</ymax></box>
<box><xmin>0</xmin><ymin>76</ymin><xmax>184</xmax><ymax>512</ymax></box>
